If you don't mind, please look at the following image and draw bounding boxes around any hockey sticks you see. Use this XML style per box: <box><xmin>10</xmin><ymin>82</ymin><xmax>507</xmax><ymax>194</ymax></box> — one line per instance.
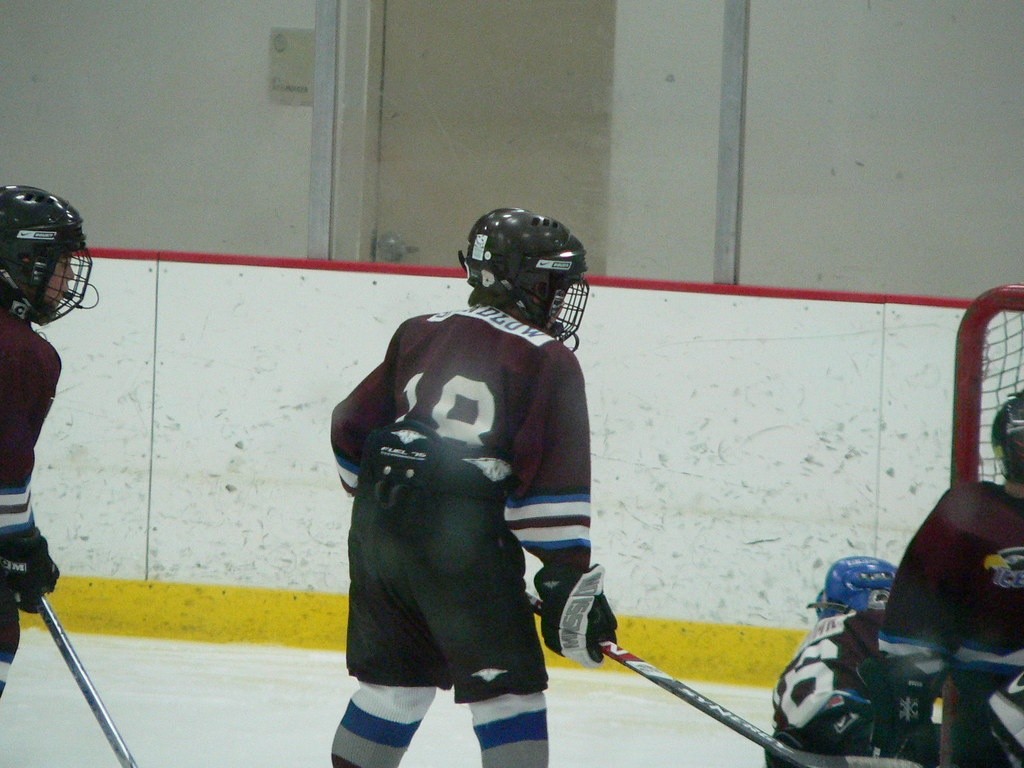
<box><xmin>34</xmin><ymin>593</ymin><xmax>144</xmax><ymax>767</ymax></box>
<box><xmin>523</xmin><ymin>590</ymin><xmax>924</xmax><ymax>768</ymax></box>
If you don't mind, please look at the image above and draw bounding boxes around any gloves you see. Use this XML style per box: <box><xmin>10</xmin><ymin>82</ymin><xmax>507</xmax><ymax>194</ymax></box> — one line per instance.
<box><xmin>0</xmin><ymin>527</ymin><xmax>60</xmax><ymax>614</ymax></box>
<box><xmin>532</xmin><ymin>563</ymin><xmax>617</xmax><ymax>667</ymax></box>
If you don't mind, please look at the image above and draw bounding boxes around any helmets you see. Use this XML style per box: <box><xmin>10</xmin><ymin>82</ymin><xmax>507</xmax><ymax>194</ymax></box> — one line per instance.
<box><xmin>812</xmin><ymin>555</ymin><xmax>897</xmax><ymax>618</ymax></box>
<box><xmin>458</xmin><ymin>208</ymin><xmax>589</xmax><ymax>342</ymax></box>
<box><xmin>0</xmin><ymin>186</ymin><xmax>93</xmax><ymax>326</ymax></box>
<box><xmin>991</xmin><ymin>396</ymin><xmax>1024</xmax><ymax>483</ymax></box>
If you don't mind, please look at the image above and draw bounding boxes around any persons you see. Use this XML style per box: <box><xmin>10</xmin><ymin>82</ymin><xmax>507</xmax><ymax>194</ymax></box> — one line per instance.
<box><xmin>872</xmin><ymin>390</ymin><xmax>1024</xmax><ymax>767</ymax></box>
<box><xmin>767</xmin><ymin>556</ymin><xmax>898</xmax><ymax>768</ymax></box>
<box><xmin>331</xmin><ymin>210</ymin><xmax>617</xmax><ymax>768</ymax></box>
<box><xmin>0</xmin><ymin>183</ymin><xmax>93</xmax><ymax>694</ymax></box>
